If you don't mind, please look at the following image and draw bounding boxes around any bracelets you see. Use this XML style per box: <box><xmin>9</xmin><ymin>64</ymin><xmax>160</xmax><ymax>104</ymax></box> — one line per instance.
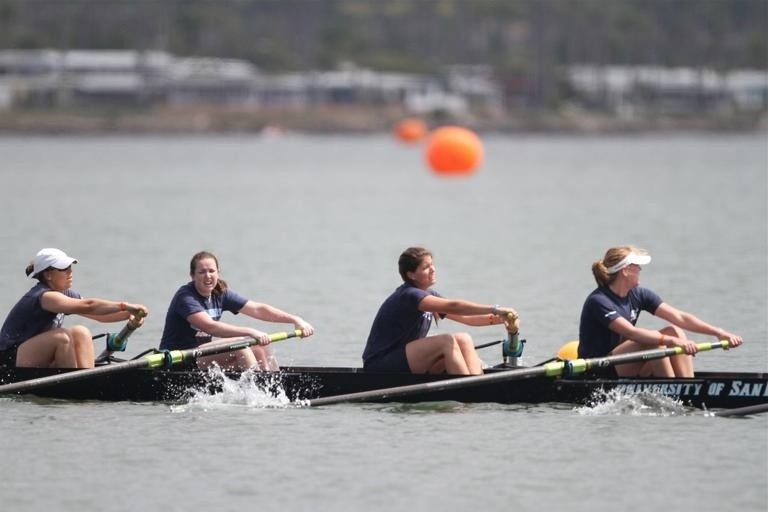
<box><xmin>493</xmin><ymin>304</ymin><xmax>499</xmax><ymax>314</ymax></box>
<box><xmin>489</xmin><ymin>314</ymin><xmax>493</xmax><ymax>324</ymax></box>
<box><xmin>660</xmin><ymin>334</ymin><xmax>665</xmax><ymax>345</ymax></box>
<box><xmin>121</xmin><ymin>302</ymin><xmax>125</xmax><ymax>311</ymax></box>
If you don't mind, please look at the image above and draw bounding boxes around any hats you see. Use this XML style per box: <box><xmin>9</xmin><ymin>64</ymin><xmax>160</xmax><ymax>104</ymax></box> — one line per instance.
<box><xmin>606</xmin><ymin>252</ymin><xmax>651</xmax><ymax>274</ymax></box>
<box><xmin>26</xmin><ymin>248</ymin><xmax>78</xmax><ymax>280</ymax></box>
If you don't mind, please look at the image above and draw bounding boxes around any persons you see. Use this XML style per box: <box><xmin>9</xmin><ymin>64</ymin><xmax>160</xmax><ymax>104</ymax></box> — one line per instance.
<box><xmin>160</xmin><ymin>251</ymin><xmax>314</xmax><ymax>372</ymax></box>
<box><xmin>0</xmin><ymin>248</ymin><xmax>149</xmax><ymax>368</ymax></box>
<box><xmin>362</xmin><ymin>248</ymin><xmax>520</xmax><ymax>375</ymax></box>
<box><xmin>578</xmin><ymin>247</ymin><xmax>744</xmax><ymax>379</ymax></box>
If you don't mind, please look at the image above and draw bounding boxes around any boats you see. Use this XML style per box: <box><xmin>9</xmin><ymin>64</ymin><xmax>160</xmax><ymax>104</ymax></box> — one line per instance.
<box><xmin>0</xmin><ymin>364</ymin><xmax>768</xmax><ymax>417</ymax></box>
